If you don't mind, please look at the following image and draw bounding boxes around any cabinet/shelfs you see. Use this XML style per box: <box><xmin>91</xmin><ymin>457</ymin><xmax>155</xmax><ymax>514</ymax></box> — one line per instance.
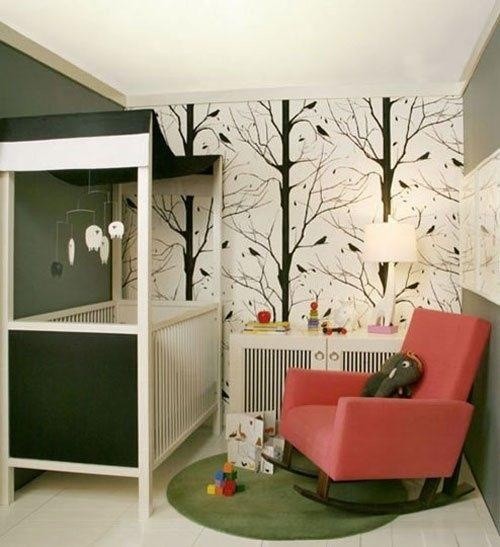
<box><xmin>230</xmin><ymin>334</ymin><xmax>404</xmax><ymax>439</ymax></box>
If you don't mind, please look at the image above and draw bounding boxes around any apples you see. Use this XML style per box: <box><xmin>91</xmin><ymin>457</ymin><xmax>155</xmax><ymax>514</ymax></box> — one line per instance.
<box><xmin>258</xmin><ymin>309</ymin><xmax>271</xmax><ymax>323</ymax></box>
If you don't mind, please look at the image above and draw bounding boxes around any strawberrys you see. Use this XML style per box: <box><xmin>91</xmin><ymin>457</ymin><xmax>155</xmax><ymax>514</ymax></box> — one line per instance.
<box><xmin>310</xmin><ymin>302</ymin><xmax>318</xmax><ymax>310</ymax></box>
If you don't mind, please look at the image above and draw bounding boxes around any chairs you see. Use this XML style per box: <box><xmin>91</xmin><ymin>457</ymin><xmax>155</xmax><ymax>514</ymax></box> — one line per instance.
<box><xmin>260</xmin><ymin>305</ymin><xmax>490</xmax><ymax>515</ymax></box>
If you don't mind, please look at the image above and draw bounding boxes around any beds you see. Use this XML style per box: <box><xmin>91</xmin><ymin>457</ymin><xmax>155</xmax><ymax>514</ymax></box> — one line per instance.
<box><xmin>2</xmin><ymin>297</ymin><xmax>224</xmax><ymax>520</ymax></box>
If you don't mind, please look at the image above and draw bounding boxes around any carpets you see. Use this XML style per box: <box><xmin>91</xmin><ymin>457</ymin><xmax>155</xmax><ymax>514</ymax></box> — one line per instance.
<box><xmin>164</xmin><ymin>444</ymin><xmax>409</xmax><ymax>539</ymax></box>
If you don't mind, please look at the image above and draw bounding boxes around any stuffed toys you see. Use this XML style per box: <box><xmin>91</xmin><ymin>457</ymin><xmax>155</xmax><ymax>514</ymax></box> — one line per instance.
<box><xmin>359</xmin><ymin>351</ymin><xmax>425</xmax><ymax>398</ymax></box>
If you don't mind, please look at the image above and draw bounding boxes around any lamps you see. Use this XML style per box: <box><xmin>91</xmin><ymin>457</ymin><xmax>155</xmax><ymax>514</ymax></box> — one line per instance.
<box><xmin>361</xmin><ymin>223</ymin><xmax>418</xmax><ymax>332</ymax></box>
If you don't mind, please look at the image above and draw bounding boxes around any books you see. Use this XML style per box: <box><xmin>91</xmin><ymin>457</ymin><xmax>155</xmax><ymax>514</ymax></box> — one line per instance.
<box><xmin>244</xmin><ymin>321</ymin><xmax>291</xmax><ymax>335</ymax></box>
